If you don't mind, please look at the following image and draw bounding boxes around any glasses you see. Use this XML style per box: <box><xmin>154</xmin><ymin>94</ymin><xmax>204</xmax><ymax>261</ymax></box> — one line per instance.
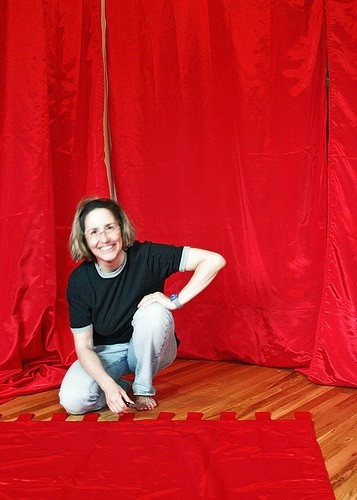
<box><xmin>84</xmin><ymin>224</ymin><xmax>121</xmax><ymax>239</ymax></box>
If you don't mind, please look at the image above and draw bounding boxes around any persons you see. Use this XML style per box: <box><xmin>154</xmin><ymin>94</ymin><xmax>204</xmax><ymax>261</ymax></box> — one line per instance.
<box><xmin>59</xmin><ymin>197</ymin><xmax>226</xmax><ymax>417</ymax></box>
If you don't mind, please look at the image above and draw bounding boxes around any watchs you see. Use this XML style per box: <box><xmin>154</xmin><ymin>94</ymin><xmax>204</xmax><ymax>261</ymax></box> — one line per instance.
<box><xmin>169</xmin><ymin>294</ymin><xmax>182</xmax><ymax>309</ymax></box>
